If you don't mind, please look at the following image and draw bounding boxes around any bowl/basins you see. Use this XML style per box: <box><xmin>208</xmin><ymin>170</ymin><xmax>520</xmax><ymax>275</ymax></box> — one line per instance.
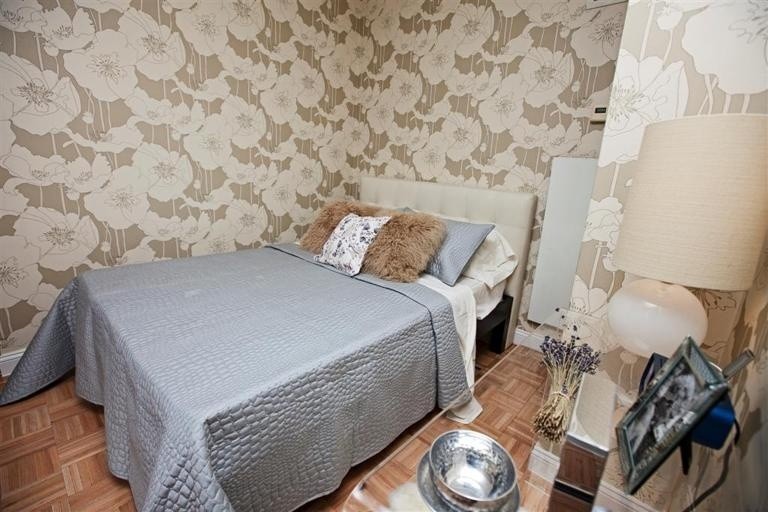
<box><xmin>427</xmin><ymin>428</ymin><xmax>520</xmax><ymax>512</ymax></box>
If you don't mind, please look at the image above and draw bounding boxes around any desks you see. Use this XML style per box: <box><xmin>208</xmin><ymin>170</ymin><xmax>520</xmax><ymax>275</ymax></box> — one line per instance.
<box><xmin>342</xmin><ymin>307</ymin><xmax>734</xmax><ymax>512</ymax></box>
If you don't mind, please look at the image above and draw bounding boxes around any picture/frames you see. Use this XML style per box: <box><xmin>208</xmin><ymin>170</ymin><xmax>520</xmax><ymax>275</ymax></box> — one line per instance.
<box><xmin>614</xmin><ymin>336</ymin><xmax>729</xmax><ymax>496</ymax></box>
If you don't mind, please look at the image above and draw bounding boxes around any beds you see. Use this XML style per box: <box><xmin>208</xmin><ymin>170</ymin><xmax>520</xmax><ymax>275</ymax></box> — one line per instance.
<box><xmin>1</xmin><ymin>175</ymin><xmax>538</xmax><ymax>511</ymax></box>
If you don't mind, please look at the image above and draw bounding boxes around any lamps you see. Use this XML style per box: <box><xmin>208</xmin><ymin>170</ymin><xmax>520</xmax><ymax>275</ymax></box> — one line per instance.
<box><xmin>607</xmin><ymin>114</ymin><xmax>768</xmax><ymax>405</ymax></box>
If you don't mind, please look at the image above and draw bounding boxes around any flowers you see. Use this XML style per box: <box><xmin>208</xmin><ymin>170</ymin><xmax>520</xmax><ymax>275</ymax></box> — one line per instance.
<box><xmin>530</xmin><ymin>308</ymin><xmax>603</xmax><ymax>444</ymax></box>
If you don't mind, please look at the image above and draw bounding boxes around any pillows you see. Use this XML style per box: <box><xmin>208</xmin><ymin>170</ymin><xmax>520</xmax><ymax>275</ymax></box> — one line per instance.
<box><xmin>360</xmin><ymin>213</ymin><xmax>448</xmax><ymax>283</ymax></box>
<box><xmin>297</xmin><ymin>198</ymin><xmax>382</xmax><ymax>255</ymax></box>
<box><xmin>314</xmin><ymin>213</ymin><xmax>392</xmax><ymax>276</ymax></box>
<box><xmin>396</xmin><ymin>207</ymin><xmax>496</xmax><ymax>286</ymax></box>
<box><xmin>412</xmin><ymin>209</ymin><xmax>519</xmax><ymax>290</ymax></box>
